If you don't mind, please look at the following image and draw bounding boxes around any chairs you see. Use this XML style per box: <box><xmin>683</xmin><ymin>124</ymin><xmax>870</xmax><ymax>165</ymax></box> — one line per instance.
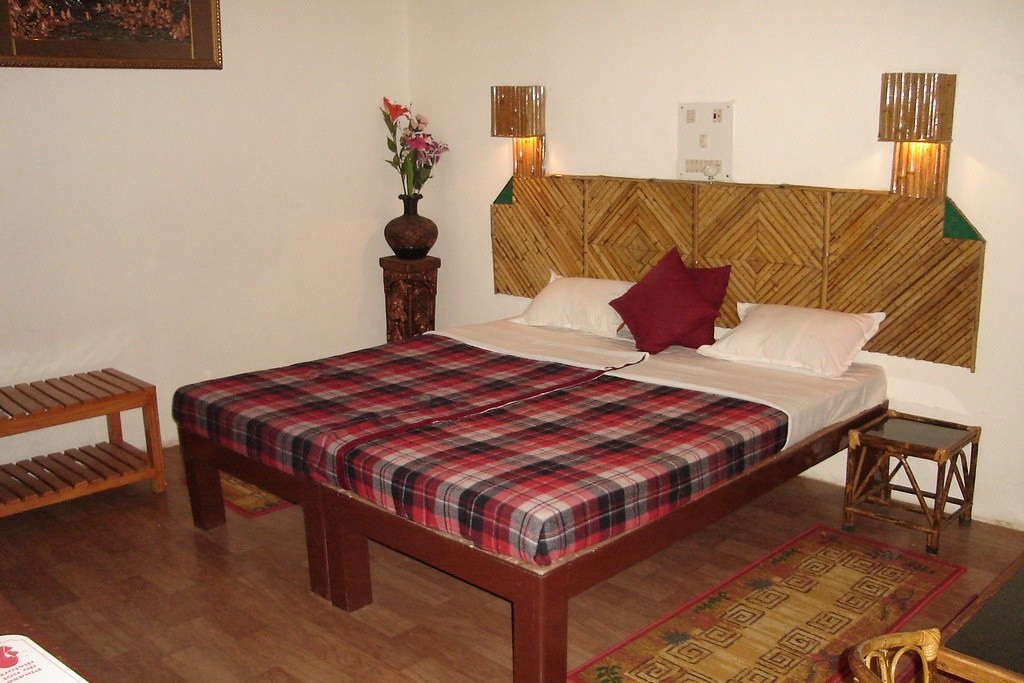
<box><xmin>849</xmin><ymin>627</ymin><xmax>1024</xmax><ymax>683</ymax></box>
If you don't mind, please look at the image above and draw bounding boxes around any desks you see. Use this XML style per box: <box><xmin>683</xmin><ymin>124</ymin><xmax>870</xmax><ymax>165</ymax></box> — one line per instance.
<box><xmin>937</xmin><ymin>552</ymin><xmax>1024</xmax><ymax>683</ymax></box>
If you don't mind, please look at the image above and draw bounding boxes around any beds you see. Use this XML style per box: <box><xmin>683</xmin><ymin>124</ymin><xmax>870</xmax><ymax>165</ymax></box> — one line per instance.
<box><xmin>174</xmin><ymin>267</ymin><xmax>889</xmax><ymax>683</ymax></box>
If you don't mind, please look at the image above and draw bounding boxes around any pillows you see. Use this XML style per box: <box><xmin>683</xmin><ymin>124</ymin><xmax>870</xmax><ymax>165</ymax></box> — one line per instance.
<box><xmin>511</xmin><ymin>269</ymin><xmax>637</xmax><ymax>344</ymax></box>
<box><xmin>673</xmin><ymin>264</ymin><xmax>734</xmax><ymax>350</ymax></box>
<box><xmin>609</xmin><ymin>246</ymin><xmax>722</xmax><ymax>357</ymax></box>
<box><xmin>696</xmin><ymin>301</ymin><xmax>886</xmax><ymax>376</ymax></box>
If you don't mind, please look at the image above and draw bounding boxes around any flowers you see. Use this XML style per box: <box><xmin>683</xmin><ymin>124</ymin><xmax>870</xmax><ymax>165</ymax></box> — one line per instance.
<box><xmin>378</xmin><ymin>95</ymin><xmax>448</xmax><ymax>199</ymax></box>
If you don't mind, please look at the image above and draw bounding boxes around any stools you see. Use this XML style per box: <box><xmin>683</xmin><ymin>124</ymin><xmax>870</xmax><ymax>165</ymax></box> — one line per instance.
<box><xmin>842</xmin><ymin>409</ymin><xmax>981</xmax><ymax>551</ymax></box>
<box><xmin>379</xmin><ymin>255</ymin><xmax>441</xmax><ymax>344</ymax></box>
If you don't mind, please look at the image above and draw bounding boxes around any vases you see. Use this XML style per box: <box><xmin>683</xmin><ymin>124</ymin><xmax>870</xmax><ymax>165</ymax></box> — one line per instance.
<box><xmin>384</xmin><ymin>193</ymin><xmax>438</xmax><ymax>260</ymax></box>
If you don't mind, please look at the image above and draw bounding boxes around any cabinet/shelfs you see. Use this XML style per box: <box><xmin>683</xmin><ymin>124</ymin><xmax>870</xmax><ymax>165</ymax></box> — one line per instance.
<box><xmin>0</xmin><ymin>367</ymin><xmax>168</xmax><ymax>520</ymax></box>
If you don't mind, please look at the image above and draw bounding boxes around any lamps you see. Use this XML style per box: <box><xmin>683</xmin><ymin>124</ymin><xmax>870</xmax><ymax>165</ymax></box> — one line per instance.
<box><xmin>878</xmin><ymin>73</ymin><xmax>956</xmax><ymax>199</ymax></box>
<box><xmin>491</xmin><ymin>86</ymin><xmax>546</xmax><ymax>177</ymax></box>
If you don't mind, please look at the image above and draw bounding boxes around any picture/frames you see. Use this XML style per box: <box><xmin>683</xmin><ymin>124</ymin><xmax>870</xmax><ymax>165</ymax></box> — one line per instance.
<box><xmin>0</xmin><ymin>0</ymin><xmax>224</xmax><ymax>70</ymax></box>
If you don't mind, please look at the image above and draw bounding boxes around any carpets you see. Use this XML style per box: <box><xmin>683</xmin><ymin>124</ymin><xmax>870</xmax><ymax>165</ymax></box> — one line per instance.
<box><xmin>566</xmin><ymin>522</ymin><xmax>966</xmax><ymax>683</ymax></box>
<box><xmin>182</xmin><ymin>469</ymin><xmax>296</xmax><ymax>520</ymax></box>
